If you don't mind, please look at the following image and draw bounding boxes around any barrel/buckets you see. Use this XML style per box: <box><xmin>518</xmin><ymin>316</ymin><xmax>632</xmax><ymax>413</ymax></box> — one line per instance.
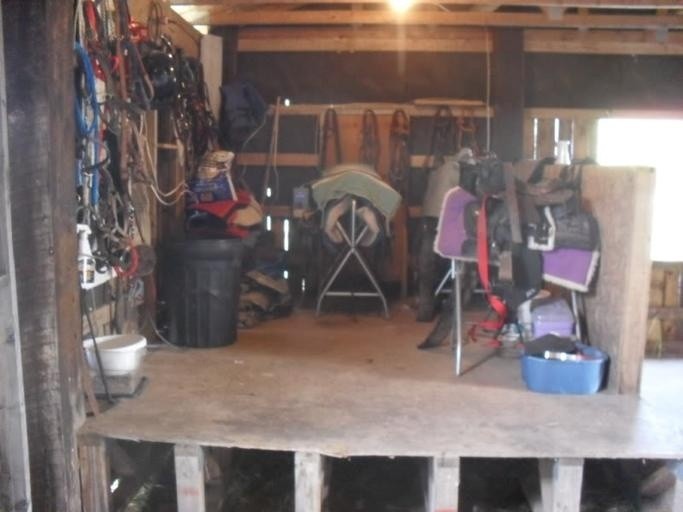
<box><xmin>160</xmin><ymin>233</ymin><xmax>245</xmax><ymax>349</ymax></box>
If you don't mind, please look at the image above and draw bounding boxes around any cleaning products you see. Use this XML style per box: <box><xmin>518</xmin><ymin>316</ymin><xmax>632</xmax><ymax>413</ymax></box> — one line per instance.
<box><xmin>76</xmin><ymin>223</ymin><xmax>95</xmax><ymax>290</ymax></box>
